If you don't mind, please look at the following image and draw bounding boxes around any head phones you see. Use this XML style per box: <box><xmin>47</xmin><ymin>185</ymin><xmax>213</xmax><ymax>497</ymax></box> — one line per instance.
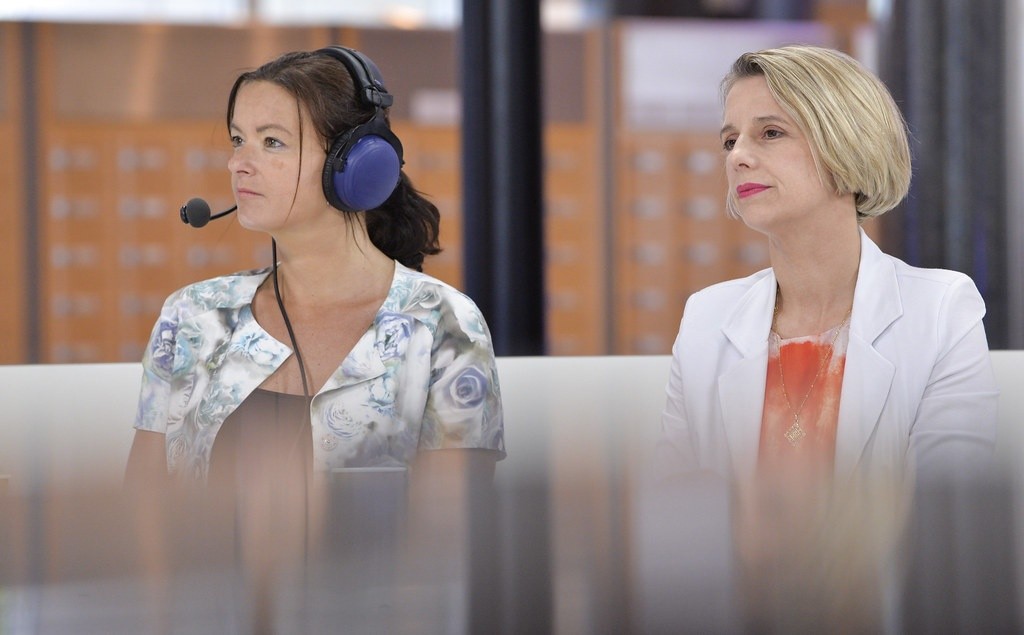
<box><xmin>309</xmin><ymin>45</ymin><xmax>406</xmax><ymax>212</ymax></box>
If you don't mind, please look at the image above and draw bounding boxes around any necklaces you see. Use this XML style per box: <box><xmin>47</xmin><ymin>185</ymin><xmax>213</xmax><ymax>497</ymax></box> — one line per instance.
<box><xmin>775</xmin><ymin>287</ymin><xmax>851</xmax><ymax>447</ymax></box>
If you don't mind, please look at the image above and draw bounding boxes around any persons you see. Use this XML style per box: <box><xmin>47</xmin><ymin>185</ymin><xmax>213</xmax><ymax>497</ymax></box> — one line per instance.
<box><xmin>640</xmin><ymin>47</ymin><xmax>999</xmax><ymax>634</ymax></box>
<box><xmin>122</xmin><ymin>53</ymin><xmax>508</xmax><ymax>635</ymax></box>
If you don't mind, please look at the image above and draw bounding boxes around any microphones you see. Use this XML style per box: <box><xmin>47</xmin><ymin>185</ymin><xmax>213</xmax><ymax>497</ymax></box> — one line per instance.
<box><xmin>179</xmin><ymin>198</ymin><xmax>238</xmax><ymax>228</ymax></box>
